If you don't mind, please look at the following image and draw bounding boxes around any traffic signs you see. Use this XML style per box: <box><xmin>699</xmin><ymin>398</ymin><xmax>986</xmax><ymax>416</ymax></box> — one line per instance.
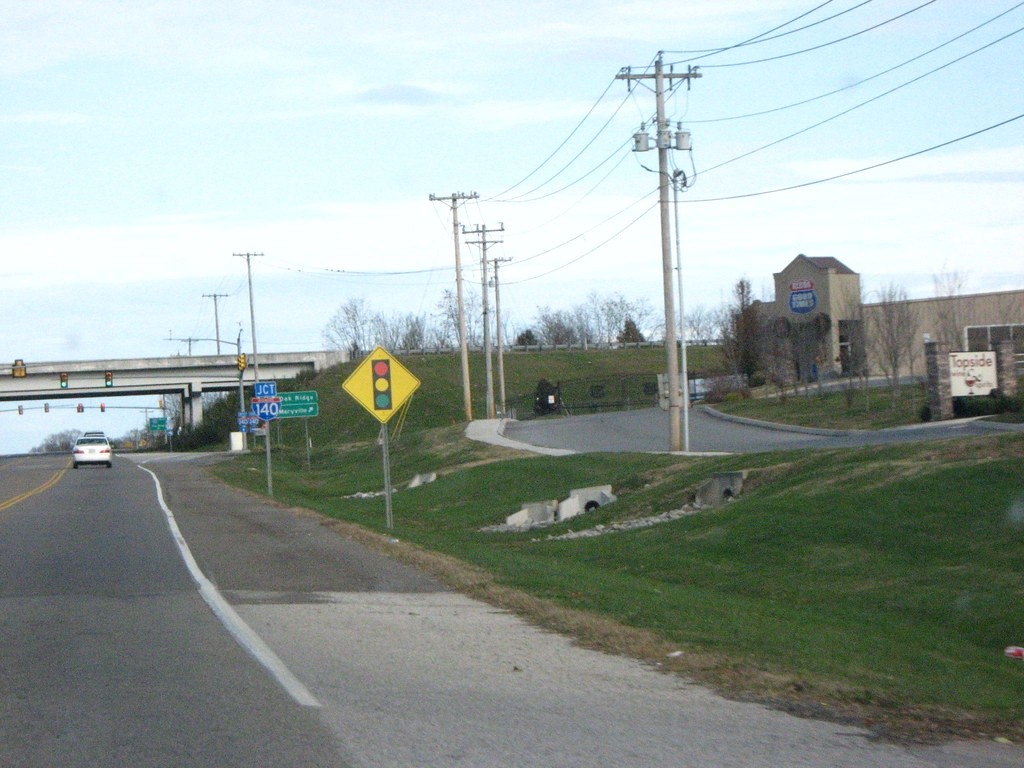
<box><xmin>150</xmin><ymin>417</ymin><xmax>168</xmax><ymax>431</ymax></box>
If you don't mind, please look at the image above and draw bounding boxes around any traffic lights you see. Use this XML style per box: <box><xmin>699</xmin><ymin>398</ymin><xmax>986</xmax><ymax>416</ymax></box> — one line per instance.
<box><xmin>105</xmin><ymin>371</ymin><xmax>112</xmax><ymax>387</ymax></box>
<box><xmin>60</xmin><ymin>372</ymin><xmax>68</xmax><ymax>388</ymax></box>
<box><xmin>238</xmin><ymin>353</ymin><xmax>247</xmax><ymax>371</ymax></box>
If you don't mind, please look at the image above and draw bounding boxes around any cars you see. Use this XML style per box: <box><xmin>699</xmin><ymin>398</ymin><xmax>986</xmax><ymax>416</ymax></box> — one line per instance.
<box><xmin>82</xmin><ymin>432</ymin><xmax>107</xmax><ymax>442</ymax></box>
<box><xmin>70</xmin><ymin>436</ymin><xmax>114</xmax><ymax>469</ymax></box>
